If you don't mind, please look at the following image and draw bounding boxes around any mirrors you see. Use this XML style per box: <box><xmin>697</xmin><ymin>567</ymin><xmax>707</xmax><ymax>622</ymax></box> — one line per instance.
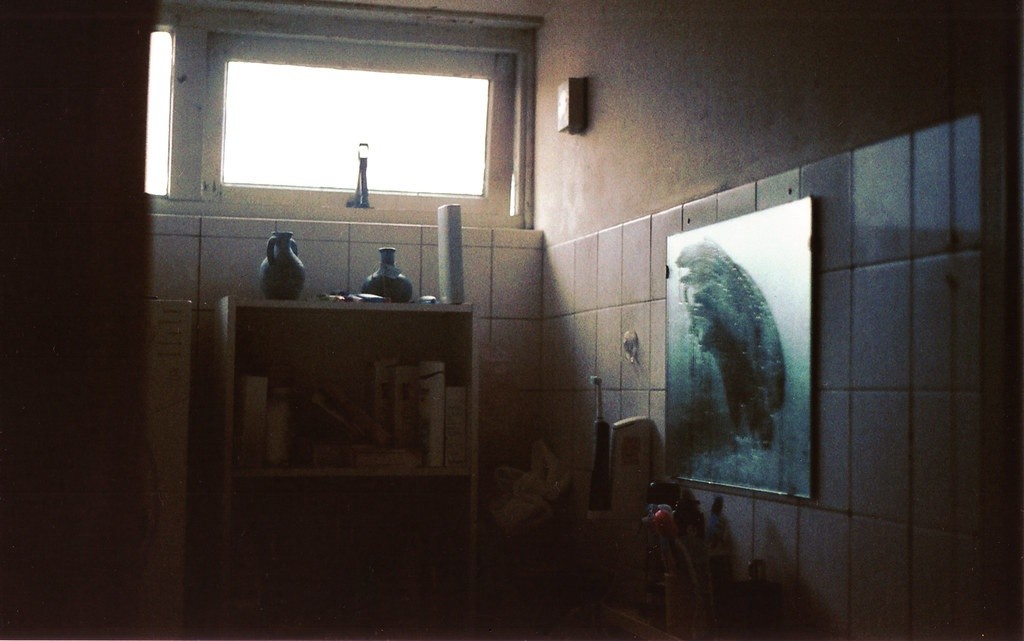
<box><xmin>660</xmin><ymin>196</ymin><xmax>818</xmax><ymax>501</ymax></box>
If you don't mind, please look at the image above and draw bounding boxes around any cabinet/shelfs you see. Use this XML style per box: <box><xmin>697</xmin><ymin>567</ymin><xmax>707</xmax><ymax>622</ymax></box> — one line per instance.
<box><xmin>212</xmin><ymin>296</ymin><xmax>478</xmax><ymax>640</ymax></box>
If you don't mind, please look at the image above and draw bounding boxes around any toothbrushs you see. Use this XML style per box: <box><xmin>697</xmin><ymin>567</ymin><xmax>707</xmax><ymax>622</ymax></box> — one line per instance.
<box><xmin>582</xmin><ymin>372</ymin><xmax>617</xmax><ymax>521</ymax></box>
<box><xmin>638</xmin><ymin>485</ymin><xmax>729</xmax><ymax>583</ymax></box>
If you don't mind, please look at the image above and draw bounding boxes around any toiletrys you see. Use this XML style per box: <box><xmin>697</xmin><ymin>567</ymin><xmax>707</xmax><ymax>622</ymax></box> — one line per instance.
<box><xmin>237</xmin><ymin>335</ymin><xmax>467</xmax><ymax>474</ymax></box>
<box><xmin>436</xmin><ymin>200</ymin><xmax>466</xmax><ymax>305</ymax></box>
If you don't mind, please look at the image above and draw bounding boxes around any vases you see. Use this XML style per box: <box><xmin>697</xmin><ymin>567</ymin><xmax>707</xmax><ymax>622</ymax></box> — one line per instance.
<box><xmin>364</xmin><ymin>246</ymin><xmax>413</xmax><ymax>304</ymax></box>
<box><xmin>261</xmin><ymin>231</ymin><xmax>305</xmax><ymax>299</ymax></box>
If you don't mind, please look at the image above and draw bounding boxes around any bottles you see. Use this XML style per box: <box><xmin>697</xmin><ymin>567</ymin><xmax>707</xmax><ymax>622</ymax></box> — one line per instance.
<box><xmin>259</xmin><ymin>232</ymin><xmax>305</xmax><ymax>299</ymax></box>
<box><xmin>362</xmin><ymin>246</ymin><xmax>413</xmax><ymax>302</ymax></box>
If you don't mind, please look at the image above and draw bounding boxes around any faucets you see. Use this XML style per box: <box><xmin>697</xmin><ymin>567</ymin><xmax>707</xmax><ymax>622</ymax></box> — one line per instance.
<box><xmin>718</xmin><ymin>555</ymin><xmax>785</xmax><ymax>641</ymax></box>
